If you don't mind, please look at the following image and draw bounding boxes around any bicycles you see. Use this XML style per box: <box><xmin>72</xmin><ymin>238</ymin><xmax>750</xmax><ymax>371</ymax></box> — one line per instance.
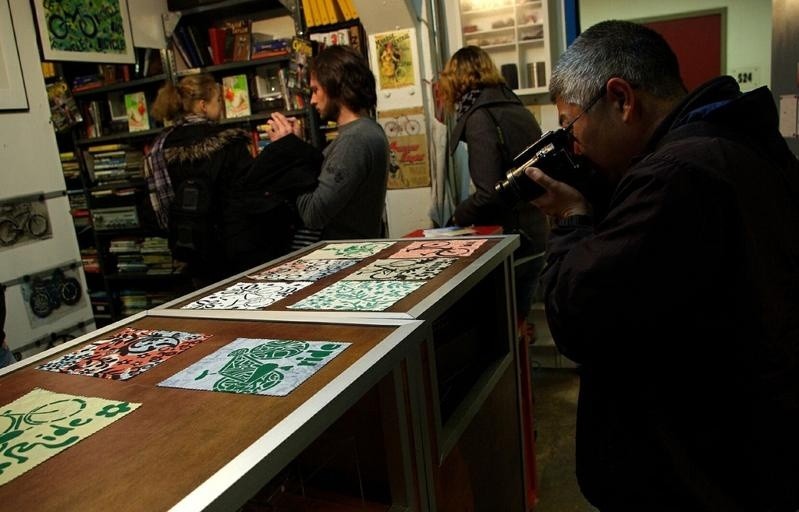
<box><xmin>384</xmin><ymin>115</ymin><xmax>420</xmax><ymax>138</ymax></box>
<box><xmin>43</xmin><ymin>0</ymin><xmax>98</xmax><ymax>39</ymax></box>
<box><xmin>395</xmin><ymin>59</ymin><xmax>407</xmax><ymax>82</ymax></box>
<box><xmin>29</xmin><ymin>268</ymin><xmax>82</xmax><ymax>318</ymax></box>
<box><xmin>0</xmin><ymin>202</ymin><xmax>48</xmax><ymax>245</ymax></box>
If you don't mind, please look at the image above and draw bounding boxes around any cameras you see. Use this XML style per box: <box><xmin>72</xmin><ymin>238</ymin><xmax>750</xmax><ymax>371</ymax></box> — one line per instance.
<box><xmin>494</xmin><ymin>128</ymin><xmax>591</xmax><ymax>203</ymax></box>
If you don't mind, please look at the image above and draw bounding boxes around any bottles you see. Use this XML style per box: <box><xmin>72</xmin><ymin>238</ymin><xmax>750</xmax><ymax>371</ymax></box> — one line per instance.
<box><xmin>527</xmin><ymin>61</ymin><xmax>548</xmax><ymax>89</ymax></box>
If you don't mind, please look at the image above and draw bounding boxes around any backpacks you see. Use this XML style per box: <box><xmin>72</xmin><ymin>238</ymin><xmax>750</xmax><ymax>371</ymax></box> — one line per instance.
<box><xmin>167</xmin><ymin>160</ymin><xmax>222</xmax><ymax>264</ymax></box>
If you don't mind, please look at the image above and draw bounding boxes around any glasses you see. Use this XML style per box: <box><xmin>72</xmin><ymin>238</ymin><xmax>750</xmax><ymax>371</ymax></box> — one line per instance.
<box><xmin>555</xmin><ymin>76</ymin><xmax>608</xmax><ymax>157</ymax></box>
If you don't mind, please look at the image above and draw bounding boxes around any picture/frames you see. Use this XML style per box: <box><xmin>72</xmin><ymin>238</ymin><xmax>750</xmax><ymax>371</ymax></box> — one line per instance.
<box><xmin>1</xmin><ymin>0</ymin><xmax>31</xmax><ymax>114</ymax></box>
<box><xmin>28</xmin><ymin>0</ymin><xmax>136</xmax><ymax>66</ymax></box>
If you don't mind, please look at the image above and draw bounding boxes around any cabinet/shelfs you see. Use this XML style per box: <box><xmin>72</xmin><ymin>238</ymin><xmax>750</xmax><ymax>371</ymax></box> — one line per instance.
<box><xmin>1</xmin><ymin>307</ymin><xmax>427</xmax><ymax>511</ymax></box>
<box><xmin>443</xmin><ymin>1</ymin><xmax>549</xmax><ymax>97</ymax></box>
<box><xmin>41</xmin><ymin>0</ymin><xmax>368</xmax><ymax>330</ymax></box>
<box><xmin>151</xmin><ymin>233</ymin><xmax>524</xmax><ymax>511</ymax></box>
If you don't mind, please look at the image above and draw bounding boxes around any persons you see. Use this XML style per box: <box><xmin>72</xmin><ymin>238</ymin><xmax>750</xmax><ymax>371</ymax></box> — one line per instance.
<box><xmin>264</xmin><ymin>44</ymin><xmax>389</xmax><ymax>251</ymax></box>
<box><xmin>141</xmin><ymin>75</ymin><xmax>273</xmax><ymax>290</ymax></box>
<box><xmin>527</xmin><ymin>20</ymin><xmax>799</xmax><ymax>512</ymax></box>
<box><xmin>439</xmin><ymin>44</ymin><xmax>546</xmax><ymax>320</ymax></box>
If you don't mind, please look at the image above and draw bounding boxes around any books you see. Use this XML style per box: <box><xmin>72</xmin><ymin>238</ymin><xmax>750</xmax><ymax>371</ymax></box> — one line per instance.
<box><xmin>42</xmin><ymin>48</ymin><xmax>189</xmax><ymax>319</ymax></box>
<box><xmin>171</xmin><ymin>20</ymin><xmax>363</xmax><ymax>157</ymax></box>
